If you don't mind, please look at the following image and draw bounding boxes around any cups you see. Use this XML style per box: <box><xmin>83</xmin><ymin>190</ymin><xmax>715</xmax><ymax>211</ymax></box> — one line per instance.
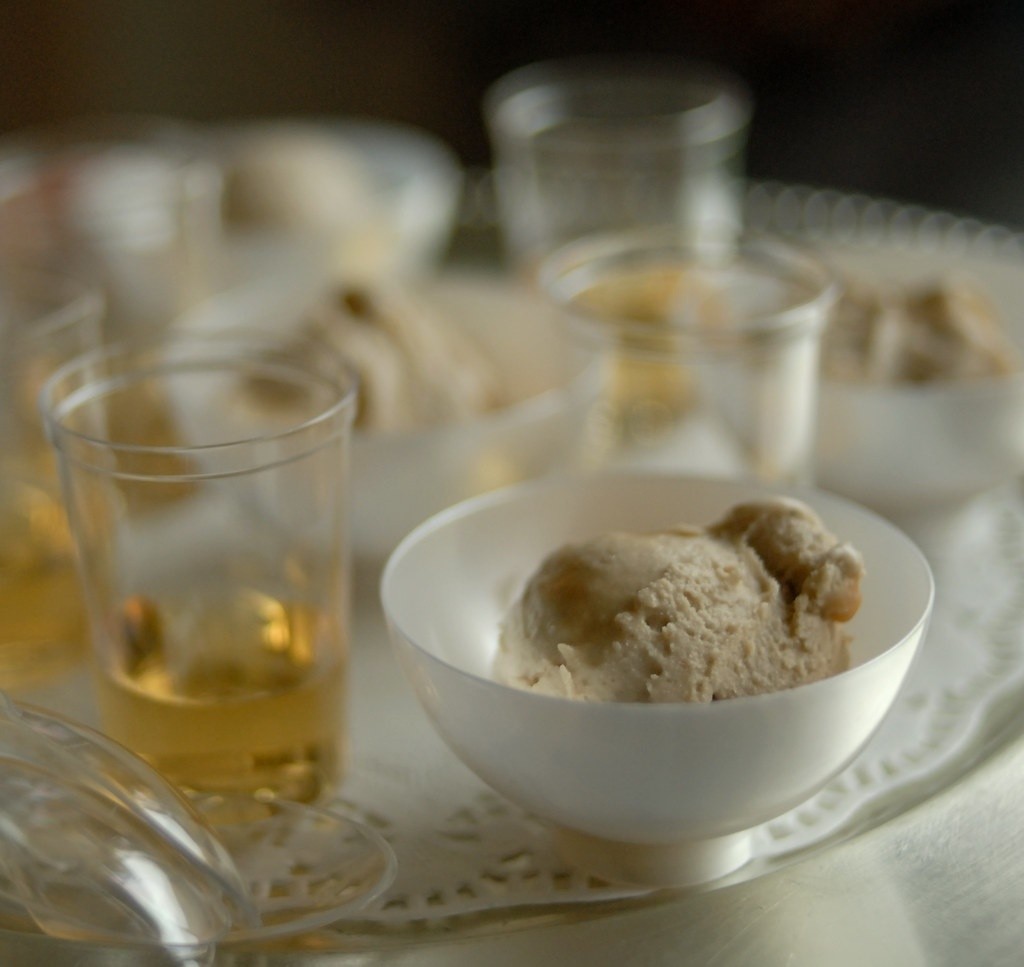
<box><xmin>537</xmin><ymin>229</ymin><xmax>838</xmax><ymax>482</ymax></box>
<box><xmin>490</xmin><ymin>67</ymin><xmax>753</xmax><ymax>273</ymax></box>
<box><xmin>34</xmin><ymin>340</ymin><xmax>357</xmax><ymax>816</ymax></box>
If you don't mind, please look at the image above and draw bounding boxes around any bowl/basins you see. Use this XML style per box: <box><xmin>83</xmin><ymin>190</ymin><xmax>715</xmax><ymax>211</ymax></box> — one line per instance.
<box><xmin>379</xmin><ymin>471</ymin><xmax>941</xmax><ymax>891</ymax></box>
